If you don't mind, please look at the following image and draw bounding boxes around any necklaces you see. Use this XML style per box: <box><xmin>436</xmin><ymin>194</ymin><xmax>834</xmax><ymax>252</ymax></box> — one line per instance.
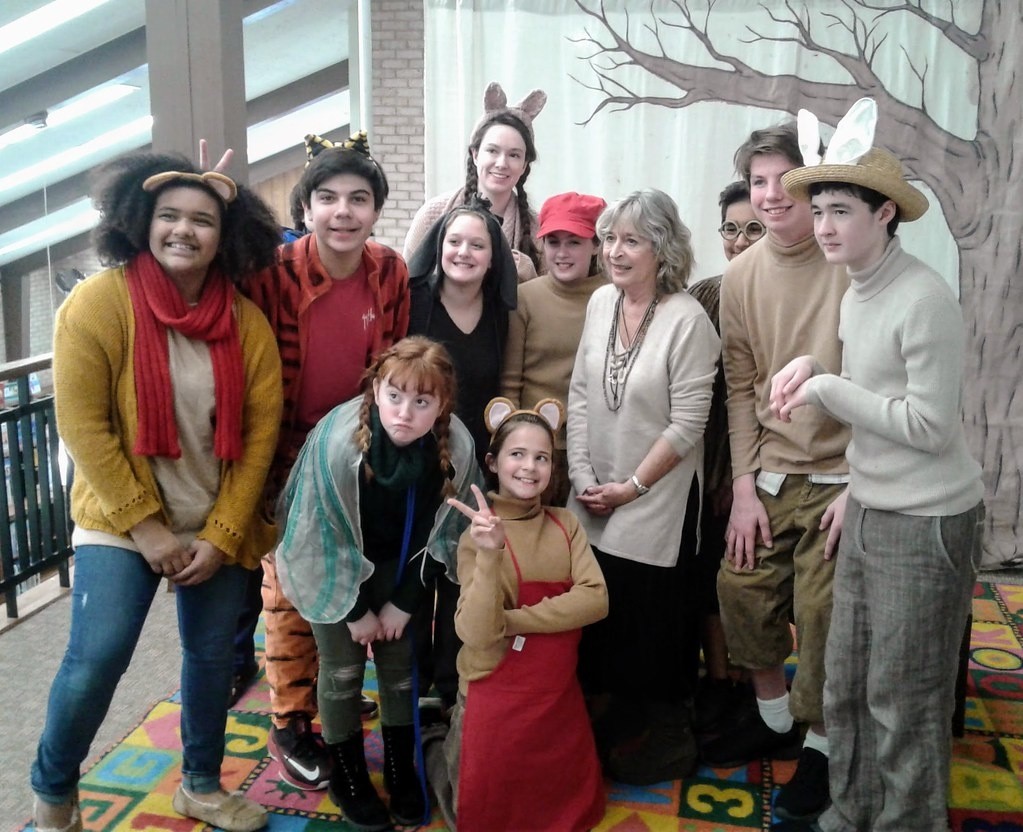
<box><xmin>603</xmin><ymin>290</ymin><xmax>660</xmax><ymax>411</ymax></box>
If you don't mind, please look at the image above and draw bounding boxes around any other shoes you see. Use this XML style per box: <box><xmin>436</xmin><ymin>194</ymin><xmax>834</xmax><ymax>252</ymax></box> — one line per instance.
<box><xmin>590</xmin><ymin>693</ymin><xmax>638</xmax><ymax>762</ymax></box>
<box><xmin>26</xmin><ymin>780</ymin><xmax>85</xmax><ymax>832</ymax></box>
<box><xmin>646</xmin><ymin>712</ymin><xmax>697</xmax><ymax>777</ymax></box>
<box><xmin>172</xmin><ymin>783</ymin><xmax>268</xmax><ymax>832</ymax></box>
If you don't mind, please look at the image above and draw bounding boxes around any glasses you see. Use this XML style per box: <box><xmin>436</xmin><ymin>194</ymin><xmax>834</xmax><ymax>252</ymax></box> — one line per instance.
<box><xmin>718</xmin><ymin>220</ymin><xmax>768</xmax><ymax>242</ymax></box>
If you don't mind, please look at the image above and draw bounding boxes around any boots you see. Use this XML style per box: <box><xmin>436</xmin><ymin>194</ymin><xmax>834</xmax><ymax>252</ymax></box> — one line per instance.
<box><xmin>322</xmin><ymin>736</ymin><xmax>391</xmax><ymax>832</ymax></box>
<box><xmin>381</xmin><ymin>725</ymin><xmax>427</xmax><ymax>826</ymax></box>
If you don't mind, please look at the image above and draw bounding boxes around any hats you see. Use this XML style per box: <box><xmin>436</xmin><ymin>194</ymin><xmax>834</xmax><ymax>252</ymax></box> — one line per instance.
<box><xmin>780</xmin><ymin>96</ymin><xmax>929</xmax><ymax>222</ymax></box>
<box><xmin>536</xmin><ymin>191</ymin><xmax>608</xmax><ymax>240</ymax></box>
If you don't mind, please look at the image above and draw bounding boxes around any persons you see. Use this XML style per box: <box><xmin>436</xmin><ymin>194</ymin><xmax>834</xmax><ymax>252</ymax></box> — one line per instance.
<box><xmin>31</xmin><ymin>153</ymin><xmax>284</xmax><ymax>832</ymax></box>
<box><xmin>686</xmin><ymin>125</ymin><xmax>853</xmax><ymax>832</ymax></box>
<box><xmin>446</xmin><ymin>397</ymin><xmax>608</xmax><ymax>832</ymax></box>
<box><xmin>199</xmin><ymin>133</ymin><xmax>409</xmax><ymax>792</ymax></box>
<box><xmin>770</xmin><ymin>98</ymin><xmax>985</xmax><ymax>832</ymax></box>
<box><xmin>684</xmin><ymin>185</ymin><xmax>769</xmax><ymax>731</ymax></box>
<box><xmin>404</xmin><ymin>80</ymin><xmax>612</xmax><ymax>724</ymax></box>
<box><xmin>563</xmin><ymin>189</ymin><xmax>721</xmax><ymax>779</ymax></box>
<box><xmin>274</xmin><ymin>339</ymin><xmax>474</xmax><ymax>832</ymax></box>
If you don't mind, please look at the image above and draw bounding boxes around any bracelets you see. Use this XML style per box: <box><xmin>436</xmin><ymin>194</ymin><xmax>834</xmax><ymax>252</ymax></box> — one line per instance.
<box><xmin>633</xmin><ymin>476</ymin><xmax>650</xmax><ymax>494</ymax></box>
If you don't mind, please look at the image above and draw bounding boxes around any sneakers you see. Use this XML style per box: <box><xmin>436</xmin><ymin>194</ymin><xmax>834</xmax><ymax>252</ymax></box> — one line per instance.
<box><xmin>264</xmin><ymin>713</ymin><xmax>331</xmax><ymax>791</ymax></box>
<box><xmin>774</xmin><ymin>740</ymin><xmax>832</xmax><ymax>823</ymax></box>
<box><xmin>359</xmin><ymin>696</ymin><xmax>378</xmax><ymax>722</ymax></box>
<box><xmin>698</xmin><ymin>705</ymin><xmax>800</xmax><ymax>768</ymax></box>
